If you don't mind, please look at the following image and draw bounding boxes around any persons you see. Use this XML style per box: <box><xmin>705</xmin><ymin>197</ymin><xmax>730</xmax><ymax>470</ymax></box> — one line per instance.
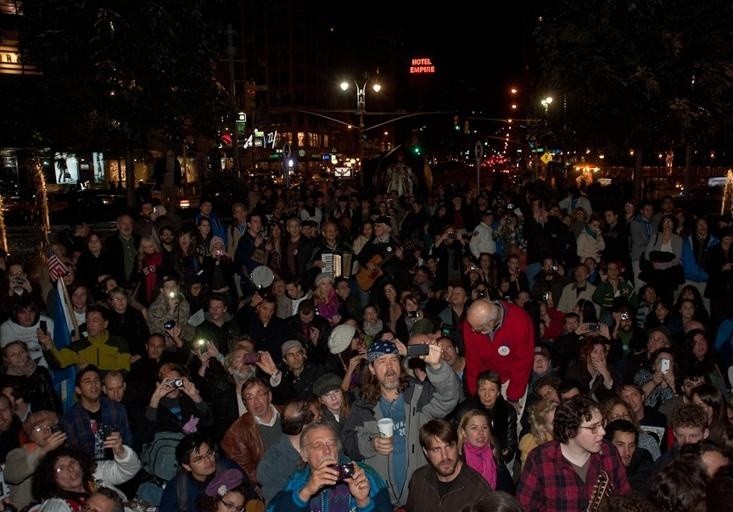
<box><xmin>0</xmin><ymin>152</ymin><xmax>733</xmax><ymax>511</ymax></box>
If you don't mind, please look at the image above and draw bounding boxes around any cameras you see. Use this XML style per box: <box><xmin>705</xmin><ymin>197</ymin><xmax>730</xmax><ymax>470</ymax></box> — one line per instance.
<box><xmin>407</xmin><ymin>310</ymin><xmax>420</xmax><ymax>318</ymax></box>
<box><xmin>470</xmin><ymin>263</ymin><xmax>477</xmax><ymax>271</ymax></box>
<box><xmin>591</xmin><ymin>325</ymin><xmax>600</xmax><ymax>330</ymax></box>
<box><xmin>327</xmin><ymin>462</ymin><xmax>354</xmax><ymax>481</ymax></box>
<box><xmin>621</xmin><ymin>312</ymin><xmax>629</xmax><ymax>320</ymax></box>
<box><xmin>448</xmin><ymin>232</ymin><xmax>457</xmax><ymax>239</ymax></box>
<box><xmin>552</xmin><ymin>265</ymin><xmax>558</xmax><ymax>271</ymax></box>
<box><xmin>477</xmin><ymin>290</ymin><xmax>486</xmax><ymax>298</ymax></box>
<box><xmin>15</xmin><ymin>277</ymin><xmax>25</xmax><ymax>285</ymax></box>
<box><xmin>164</xmin><ymin>378</ymin><xmax>183</xmax><ymax>392</ymax></box>
<box><xmin>243</xmin><ymin>352</ymin><xmax>261</xmax><ymax>364</ymax></box>
<box><xmin>164</xmin><ymin>320</ymin><xmax>174</xmax><ymax>329</ymax></box>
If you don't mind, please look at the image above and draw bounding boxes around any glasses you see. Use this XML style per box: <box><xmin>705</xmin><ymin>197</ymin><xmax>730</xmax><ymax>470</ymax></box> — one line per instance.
<box><xmin>216</xmin><ymin>495</ymin><xmax>244</xmax><ymax>512</ymax></box>
<box><xmin>580</xmin><ymin>419</ymin><xmax>607</xmax><ymax>434</ymax></box>
<box><xmin>192</xmin><ymin>449</ymin><xmax>213</xmax><ymax>464</ymax></box>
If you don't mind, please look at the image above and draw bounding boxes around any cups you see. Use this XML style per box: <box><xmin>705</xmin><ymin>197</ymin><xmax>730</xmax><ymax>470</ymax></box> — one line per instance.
<box><xmin>376</xmin><ymin>418</ymin><xmax>394</xmax><ymax>441</ymax></box>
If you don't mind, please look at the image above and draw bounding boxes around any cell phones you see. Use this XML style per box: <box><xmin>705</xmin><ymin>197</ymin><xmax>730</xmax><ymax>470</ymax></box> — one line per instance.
<box><xmin>200</xmin><ymin>339</ymin><xmax>207</xmax><ymax>355</ymax></box>
<box><xmin>407</xmin><ymin>344</ymin><xmax>429</xmax><ymax>356</ymax></box>
<box><xmin>104</xmin><ymin>425</ymin><xmax>114</xmax><ymax>440</ymax></box>
<box><xmin>40</xmin><ymin>320</ymin><xmax>47</xmax><ymax>336</ymax></box>
<box><xmin>52</xmin><ymin>425</ymin><xmax>62</xmax><ymax>432</ymax></box>
<box><xmin>660</xmin><ymin>359</ymin><xmax>671</xmax><ymax>373</ymax></box>
<box><xmin>542</xmin><ymin>292</ymin><xmax>548</xmax><ymax>303</ymax></box>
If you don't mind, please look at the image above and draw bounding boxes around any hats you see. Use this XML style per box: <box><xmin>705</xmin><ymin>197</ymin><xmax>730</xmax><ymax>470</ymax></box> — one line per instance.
<box><xmin>205</xmin><ymin>469</ymin><xmax>243</xmax><ymax>496</ymax></box>
<box><xmin>312</xmin><ymin>373</ymin><xmax>341</xmax><ymax>396</ymax></box>
<box><xmin>210</xmin><ymin>235</ymin><xmax>224</xmax><ymax>252</ymax></box>
<box><xmin>315</xmin><ymin>273</ymin><xmax>335</xmax><ymax>288</ymax></box>
<box><xmin>534</xmin><ymin>346</ymin><xmax>549</xmax><ymax>357</ymax></box>
<box><xmin>23</xmin><ymin>411</ymin><xmax>59</xmax><ymax>432</ymax></box>
<box><xmin>282</xmin><ymin>340</ymin><xmax>308</xmax><ymax>364</ymax></box>
<box><xmin>367</xmin><ymin>340</ymin><xmax>401</xmax><ymax>362</ymax></box>
<box><xmin>328</xmin><ymin>325</ymin><xmax>356</xmax><ymax>355</ymax></box>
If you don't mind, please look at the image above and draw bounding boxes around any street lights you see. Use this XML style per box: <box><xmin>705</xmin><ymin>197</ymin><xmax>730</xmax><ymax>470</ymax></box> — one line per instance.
<box><xmin>336</xmin><ymin>70</ymin><xmax>382</xmax><ymax>161</ymax></box>
<box><xmin>538</xmin><ymin>95</ymin><xmax>553</xmax><ymax>179</ymax></box>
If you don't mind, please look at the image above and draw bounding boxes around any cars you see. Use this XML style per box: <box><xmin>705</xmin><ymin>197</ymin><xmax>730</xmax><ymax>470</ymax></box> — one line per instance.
<box><xmin>3</xmin><ymin>171</ymin><xmax>277</xmax><ymax>223</ymax></box>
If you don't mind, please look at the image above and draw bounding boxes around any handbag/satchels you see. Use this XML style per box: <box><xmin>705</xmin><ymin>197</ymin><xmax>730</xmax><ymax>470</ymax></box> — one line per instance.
<box><xmin>139</xmin><ymin>430</ymin><xmax>184</xmax><ymax>482</ymax></box>
<box><xmin>639</xmin><ymin>250</ymin><xmax>650</xmax><ymax>272</ymax></box>
